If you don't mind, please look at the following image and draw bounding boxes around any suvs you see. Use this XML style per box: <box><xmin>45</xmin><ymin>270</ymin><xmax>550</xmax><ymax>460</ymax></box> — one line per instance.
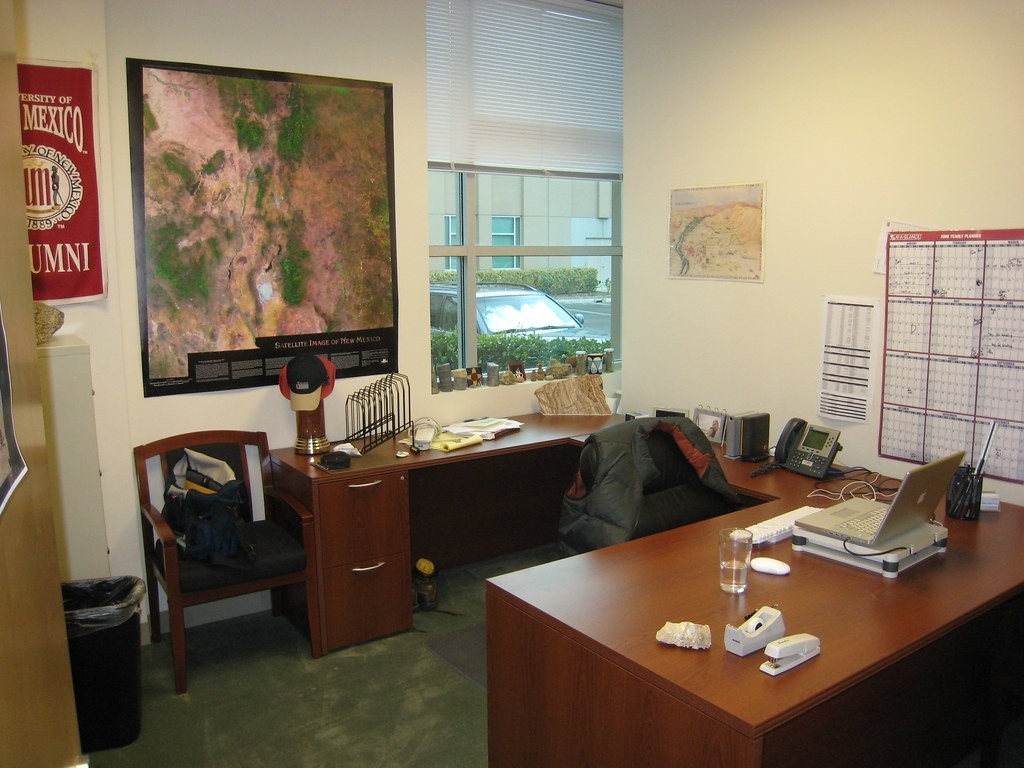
<box><xmin>431</xmin><ymin>282</ymin><xmax>611</xmax><ymax>343</ymax></box>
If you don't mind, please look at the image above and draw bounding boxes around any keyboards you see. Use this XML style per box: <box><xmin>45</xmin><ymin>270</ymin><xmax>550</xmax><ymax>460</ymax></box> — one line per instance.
<box><xmin>730</xmin><ymin>506</ymin><xmax>824</xmax><ymax>544</ymax></box>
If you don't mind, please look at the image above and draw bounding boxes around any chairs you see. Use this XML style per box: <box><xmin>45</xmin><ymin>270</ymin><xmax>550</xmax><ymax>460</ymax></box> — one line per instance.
<box><xmin>133</xmin><ymin>429</ymin><xmax>323</xmax><ymax>695</ymax></box>
<box><xmin>579</xmin><ymin>429</ymin><xmax>740</xmax><ymax>539</ymax></box>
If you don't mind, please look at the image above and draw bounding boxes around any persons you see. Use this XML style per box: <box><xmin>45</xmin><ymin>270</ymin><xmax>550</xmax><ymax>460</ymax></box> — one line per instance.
<box><xmin>707</xmin><ymin>420</ymin><xmax>721</xmax><ymax>438</ymax></box>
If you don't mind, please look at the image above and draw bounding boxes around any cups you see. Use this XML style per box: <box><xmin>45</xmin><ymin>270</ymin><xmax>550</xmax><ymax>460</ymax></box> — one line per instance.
<box><xmin>588</xmin><ymin>354</ymin><xmax>603</xmax><ymax>374</ymax></box>
<box><xmin>719</xmin><ymin>528</ymin><xmax>753</xmax><ymax>594</ymax></box>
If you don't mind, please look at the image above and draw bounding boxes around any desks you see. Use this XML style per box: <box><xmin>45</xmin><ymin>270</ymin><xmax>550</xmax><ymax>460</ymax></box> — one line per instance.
<box><xmin>484</xmin><ymin>472</ymin><xmax>1024</xmax><ymax>768</ymax></box>
<box><xmin>266</xmin><ymin>413</ymin><xmax>628</xmax><ymax>655</ymax></box>
<box><xmin>567</xmin><ymin>433</ymin><xmax>866</xmax><ymax>501</ymax></box>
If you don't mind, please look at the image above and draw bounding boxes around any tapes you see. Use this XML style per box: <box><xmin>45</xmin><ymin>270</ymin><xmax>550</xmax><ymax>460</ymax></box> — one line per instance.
<box><xmin>746</xmin><ymin>617</ymin><xmax>765</xmax><ymax>634</ymax></box>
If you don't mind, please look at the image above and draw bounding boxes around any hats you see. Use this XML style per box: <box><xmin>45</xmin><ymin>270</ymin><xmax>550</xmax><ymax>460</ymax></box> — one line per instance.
<box><xmin>287</xmin><ymin>354</ymin><xmax>326</xmax><ymax>411</ymax></box>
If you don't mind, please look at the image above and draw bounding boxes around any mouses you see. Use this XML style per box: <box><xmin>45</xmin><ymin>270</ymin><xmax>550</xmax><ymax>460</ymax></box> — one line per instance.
<box><xmin>751</xmin><ymin>557</ymin><xmax>791</xmax><ymax>575</ymax></box>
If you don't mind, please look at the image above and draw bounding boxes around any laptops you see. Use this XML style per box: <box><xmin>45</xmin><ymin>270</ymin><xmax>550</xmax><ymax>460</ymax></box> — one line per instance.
<box><xmin>795</xmin><ymin>451</ymin><xmax>965</xmax><ymax>545</ymax></box>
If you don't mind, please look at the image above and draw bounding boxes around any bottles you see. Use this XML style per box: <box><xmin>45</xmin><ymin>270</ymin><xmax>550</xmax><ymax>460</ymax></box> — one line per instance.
<box><xmin>531</xmin><ymin>370</ymin><xmax>536</xmax><ymax>381</ymax></box>
<box><xmin>604</xmin><ymin>348</ymin><xmax>614</xmax><ymax>373</ymax></box>
<box><xmin>576</xmin><ymin>351</ymin><xmax>587</xmax><ymax>376</ymax></box>
<box><xmin>537</xmin><ymin>361</ymin><xmax>544</xmax><ymax>380</ymax></box>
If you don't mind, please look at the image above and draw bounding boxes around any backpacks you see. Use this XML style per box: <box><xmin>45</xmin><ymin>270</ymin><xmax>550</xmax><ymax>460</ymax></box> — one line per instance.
<box><xmin>159</xmin><ymin>447</ymin><xmax>257</xmax><ymax>564</ymax></box>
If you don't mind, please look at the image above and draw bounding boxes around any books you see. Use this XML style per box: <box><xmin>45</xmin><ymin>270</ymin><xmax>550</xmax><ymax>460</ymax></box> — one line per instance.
<box><xmin>447</xmin><ymin>427</ymin><xmax>520</xmax><ymax>439</ymax></box>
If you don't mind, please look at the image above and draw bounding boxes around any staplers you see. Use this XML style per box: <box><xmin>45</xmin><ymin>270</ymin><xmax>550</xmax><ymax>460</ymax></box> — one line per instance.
<box><xmin>757</xmin><ymin>631</ymin><xmax>819</xmax><ymax>676</ymax></box>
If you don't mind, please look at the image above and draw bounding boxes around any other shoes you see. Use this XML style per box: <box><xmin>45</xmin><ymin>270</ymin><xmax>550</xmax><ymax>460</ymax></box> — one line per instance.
<box><xmin>411</xmin><ymin>551</ymin><xmax>462</xmax><ymax>632</ymax></box>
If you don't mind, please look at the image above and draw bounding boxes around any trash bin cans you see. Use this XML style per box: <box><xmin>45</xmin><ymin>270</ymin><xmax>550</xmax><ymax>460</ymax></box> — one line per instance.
<box><xmin>61</xmin><ymin>575</ymin><xmax>147</xmax><ymax>754</ymax></box>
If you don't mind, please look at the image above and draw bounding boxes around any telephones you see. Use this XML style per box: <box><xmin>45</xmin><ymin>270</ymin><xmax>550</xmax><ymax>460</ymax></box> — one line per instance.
<box><xmin>774</xmin><ymin>418</ymin><xmax>841</xmax><ymax>481</ymax></box>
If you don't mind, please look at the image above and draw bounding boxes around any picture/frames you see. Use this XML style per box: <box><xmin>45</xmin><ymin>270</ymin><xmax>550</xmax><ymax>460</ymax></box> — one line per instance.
<box><xmin>652</xmin><ymin>407</ymin><xmax>689</xmax><ymax>418</ymax></box>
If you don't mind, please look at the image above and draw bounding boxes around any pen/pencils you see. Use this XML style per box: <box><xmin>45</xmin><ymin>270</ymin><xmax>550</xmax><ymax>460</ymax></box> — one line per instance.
<box><xmin>463</xmin><ymin>416</ymin><xmax>488</xmax><ymax>423</ymax></box>
<box><xmin>744</xmin><ymin>603</ymin><xmax>779</xmax><ymax>621</ymax></box>
<box><xmin>948</xmin><ymin>464</ymin><xmax>979</xmax><ymax>520</ymax></box>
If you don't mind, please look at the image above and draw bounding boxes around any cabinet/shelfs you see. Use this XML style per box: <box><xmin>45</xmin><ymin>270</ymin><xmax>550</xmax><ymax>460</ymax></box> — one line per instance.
<box><xmin>37</xmin><ymin>334</ymin><xmax>111</xmax><ymax>583</ymax></box>
